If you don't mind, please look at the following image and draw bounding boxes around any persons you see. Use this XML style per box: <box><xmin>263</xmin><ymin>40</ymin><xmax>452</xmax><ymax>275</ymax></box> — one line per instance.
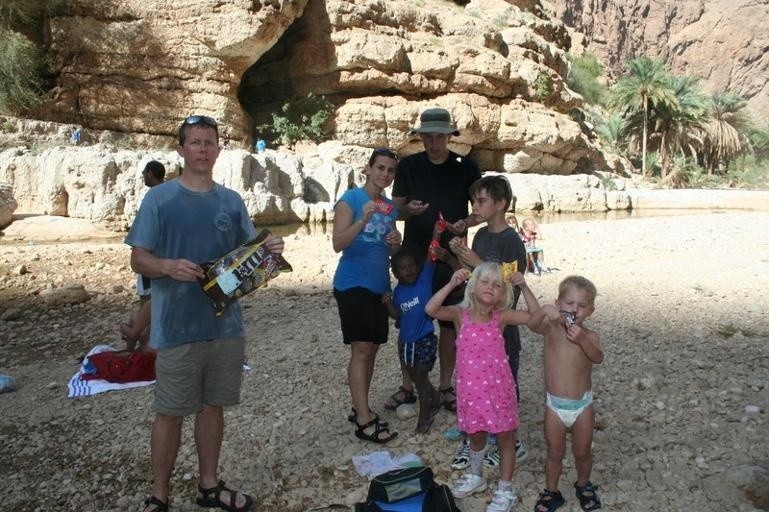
<box><xmin>423</xmin><ymin>262</ymin><xmax>540</xmax><ymax>510</ymax></box>
<box><xmin>129</xmin><ymin>116</ymin><xmax>283</xmax><ymax>511</ymax></box>
<box><xmin>120</xmin><ymin>299</ymin><xmax>152</xmax><ymax>348</ymax></box>
<box><xmin>382</xmin><ymin>100</ymin><xmax>542</xmax><ymax>434</ymax></box>
<box><xmin>330</xmin><ymin>148</ymin><xmax>402</xmax><ymax>442</ymax></box>
<box><xmin>139</xmin><ymin>161</ymin><xmax>170</xmax><ymax>309</ymax></box>
<box><xmin>527</xmin><ymin>274</ymin><xmax>605</xmax><ymax>510</ymax></box>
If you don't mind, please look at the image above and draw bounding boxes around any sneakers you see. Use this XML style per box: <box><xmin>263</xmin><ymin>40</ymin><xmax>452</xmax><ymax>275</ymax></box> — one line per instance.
<box><xmin>445</xmin><ymin>423</ymin><xmax>527</xmax><ymax>511</ymax></box>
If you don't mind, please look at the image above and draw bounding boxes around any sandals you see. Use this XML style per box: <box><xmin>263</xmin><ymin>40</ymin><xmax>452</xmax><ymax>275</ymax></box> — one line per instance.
<box><xmin>349</xmin><ymin>384</ymin><xmax>456</xmax><ymax>444</ymax></box>
<box><xmin>142</xmin><ymin>495</ymin><xmax>169</xmax><ymax>512</ymax></box>
<box><xmin>534</xmin><ymin>489</ymin><xmax>564</xmax><ymax>512</ymax></box>
<box><xmin>195</xmin><ymin>482</ymin><xmax>251</xmax><ymax>511</ymax></box>
<box><xmin>575</xmin><ymin>480</ymin><xmax>600</xmax><ymax>511</ymax></box>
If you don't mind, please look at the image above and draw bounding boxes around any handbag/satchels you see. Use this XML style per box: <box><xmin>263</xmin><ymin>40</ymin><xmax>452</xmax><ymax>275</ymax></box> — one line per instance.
<box><xmin>82</xmin><ymin>350</ymin><xmax>155</xmax><ymax>382</ymax></box>
<box><xmin>355</xmin><ymin>467</ymin><xmax>461</xmax><ymax>512</ymax></box>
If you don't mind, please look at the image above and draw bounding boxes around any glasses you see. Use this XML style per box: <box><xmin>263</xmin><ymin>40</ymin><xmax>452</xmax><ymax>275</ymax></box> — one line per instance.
<box><xmin>375</xmin><ymin>147</ymin><xmax>398</xmax><ymax>159</ymax></box>
<box><xmin>186</xmin><ymin>116</ymin><xmax>216</xmax><ymax>126</ymax></box>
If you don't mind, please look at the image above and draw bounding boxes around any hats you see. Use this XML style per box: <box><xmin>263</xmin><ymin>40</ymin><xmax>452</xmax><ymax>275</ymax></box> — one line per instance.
<box><xmin>411</xmin><ymin>108</ymin><xmax>460</xmax><ymax>137</ymax></box>
